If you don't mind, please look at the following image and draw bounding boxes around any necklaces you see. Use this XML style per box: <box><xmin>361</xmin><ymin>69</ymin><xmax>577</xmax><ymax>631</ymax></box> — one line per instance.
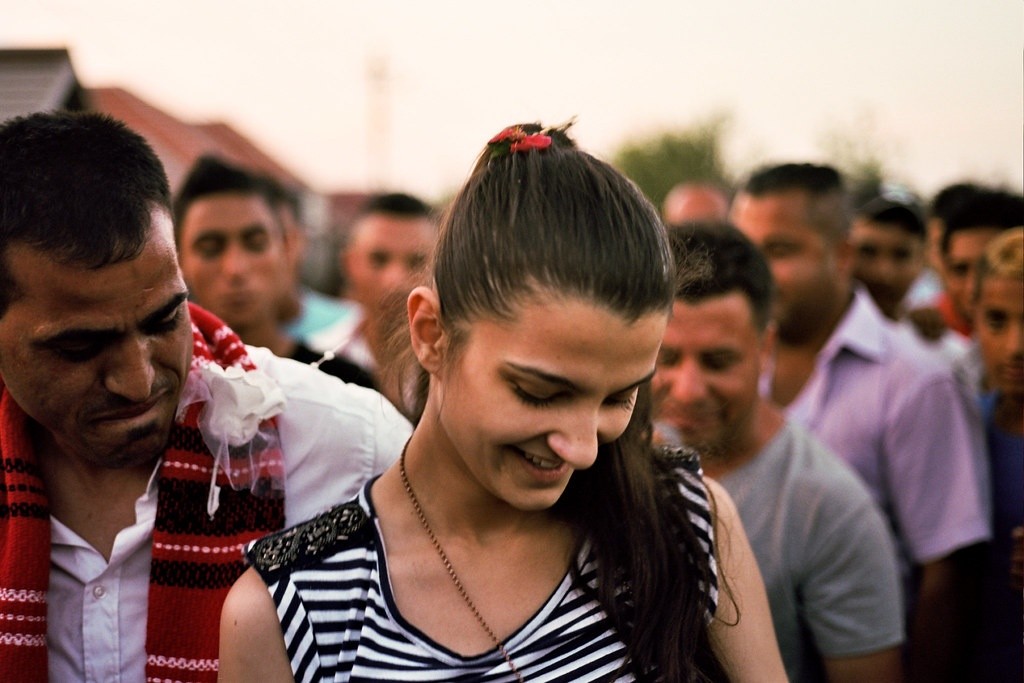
<box><xmin>399</xmin><ymin>437</ymin><xmax>526</xmax><ymax>683</ymax></box>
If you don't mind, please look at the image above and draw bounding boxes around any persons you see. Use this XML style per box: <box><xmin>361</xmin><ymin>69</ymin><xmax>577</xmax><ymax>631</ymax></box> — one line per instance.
<box><xmin>0</xmin><ymin>113</ymin><xmax>1024</xmax><ymax>683</ymax></box>
